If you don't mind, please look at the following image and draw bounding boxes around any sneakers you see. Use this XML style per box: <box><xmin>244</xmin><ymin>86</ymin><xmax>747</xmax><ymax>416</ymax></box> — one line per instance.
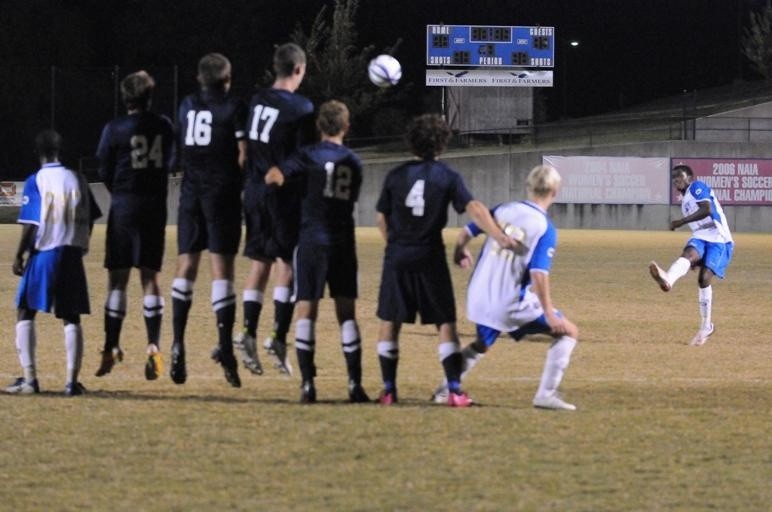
<box><xmin>689</xmin><ymin>321</ymin><xmax>715</xmax><ymax>346</ymax></box>
<box><xmin>430</xmin><ymin>386</ymin><xmax>451</xmax><ymax>404</ymax></box>
<box><xmin>262</xmin><ymin>336</ymin><xmax>292</xmax><ymax>376</ymax></box>
<box><xmin>447</xmin><ymin>391</ymin><xmax>473</xmax><ymax>408</ymax></box>
<box><xmin>170</xmin><ymin>342</ymin><xmax>188</xmax><ymax>385</ymax></box>
<box><xmin>209</xmin><ymin>344</ymin><xmax>242</xmax><ymax>388</ymax></box>
<box><xmin>532</xmin><ymin>392</ymin><xmax>576</xmax><ymax>411</ymax></box>
<box><xmin>647</xmin><ymin>260</ymin><xmax>672</xmax><ymax>293</ymax></box>
<box><xmin>299</xmin><ymin>381</ymin><xmax>317</xmax><ymax>402</ymax></box>
<box><xmin>232</xmin><ymin>332</ymin><xmax>264</xmax><ymax>376</ymax></box>
<box><xmin>94</xmin><ymin>346</ymin><xmax>124</xmax><ymax>377</ymax></box>
<box><xmin>65</xmin><ymin>382</ymin><xmax>86</xmax><ymax>398</ymax></box>
<box><xmin>143</xmin><ymin>343</ymin><xmax>162</xmax><ymax>381</ymax></box>
<box><xmin>0</xmin><ymin>376</ymin><xmax>40</xmax><ymax>394</ymax></box>
<box><xmin>378</xmin><ymin>391</ymin><xmax>398</xmax><ymax>406</ymax></box>
<box><xmin>346</xmin><ymin>382</ymin><xmax>371</xmax><ymax>402</ymax></box>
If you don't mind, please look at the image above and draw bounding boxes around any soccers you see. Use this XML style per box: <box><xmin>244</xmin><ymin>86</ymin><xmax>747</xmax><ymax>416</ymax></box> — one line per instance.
<box><xmin>368</xmin><ymin>54</ymin><xmax>402</xmax><ymax>88</ymax></box>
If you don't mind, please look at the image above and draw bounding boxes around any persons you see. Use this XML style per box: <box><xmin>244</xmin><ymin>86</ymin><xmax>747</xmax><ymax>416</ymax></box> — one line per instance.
<box><xmin>164</xmin><ymin>50</ymin><xmax>245</xmax><ymax>387</ymax></box>
<box><xmin>233</xmin><ymin>43</ymin><xmax>318</xmax><ymax>380</ymax></box>
<box><xmin>428</xmin><ymin>164</ymin><xmax>581</xmax><ymax>413</ymax></box>
<box><xmin>373</xmin><ymin>114</ymin><xmax>527</xmax><ymax>407</ymax></box>
<box><xmin>648</xmin><ymin>164</ymin><xmax>735</xmax><ymax>347</ymax></box>
<box><xmin>265</xmin><ymin>98</ymin><xmax>372</xmax><ymax>403</ymax></box>
<box><xmin>98</xmin><ymin>71</ymin><xmax>177</xmax><ymax>380</ymax></box>
<box><xmin>0</xmin><ymin>131</ymin><xmax>102</xmax><ymax>397</ymax></box>
